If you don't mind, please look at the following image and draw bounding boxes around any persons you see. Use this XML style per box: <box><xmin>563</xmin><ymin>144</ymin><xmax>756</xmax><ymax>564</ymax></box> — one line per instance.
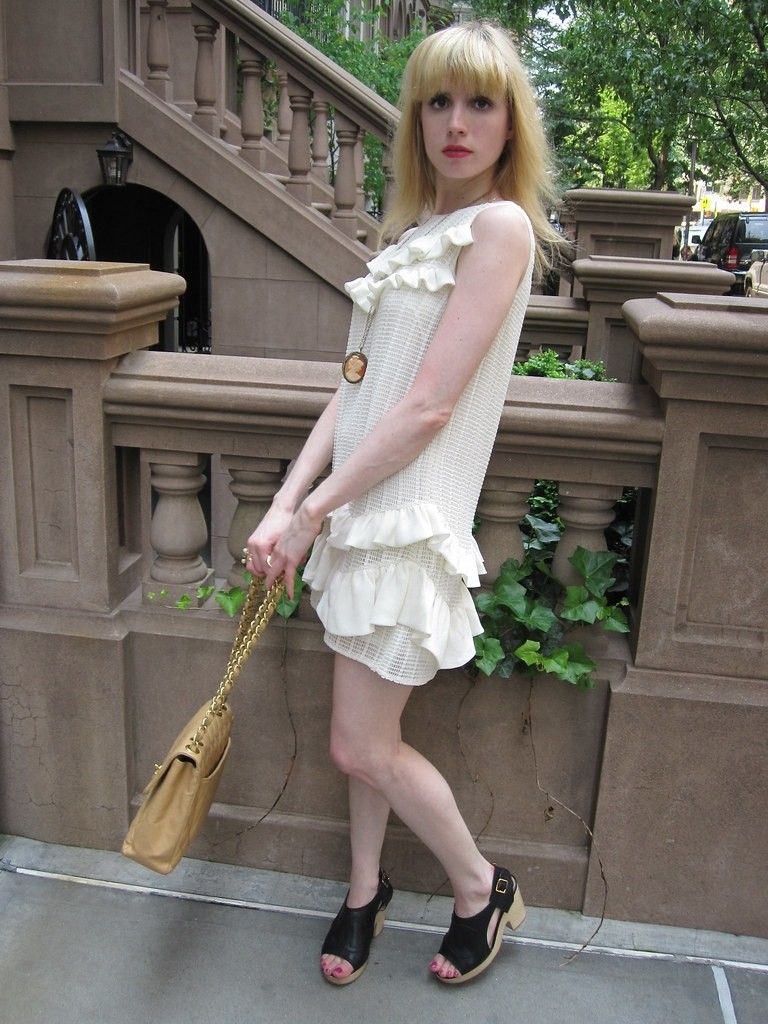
<box><xmin>246</xmin><ymin>23</ymin><xmax>561</xmax><ymax>986</ymax></box>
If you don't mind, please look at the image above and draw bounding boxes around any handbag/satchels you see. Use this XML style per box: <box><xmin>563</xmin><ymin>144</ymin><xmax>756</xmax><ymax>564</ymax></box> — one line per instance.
<box><xmin>121</xmin><ymin>571</ymin><xmax>284</xmax><ymax>875</ymax></box>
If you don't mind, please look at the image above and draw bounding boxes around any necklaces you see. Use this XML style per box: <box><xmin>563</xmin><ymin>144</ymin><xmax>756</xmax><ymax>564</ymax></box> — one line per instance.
<box><xmin>341</xmin><ymin>189</ymin><xmax>492</xmax><ymax>384</ymax></box>
<box><xmin>488</xmin><ymin>197</ymin><xmax>497</xmax><ymax>202</ymax></box>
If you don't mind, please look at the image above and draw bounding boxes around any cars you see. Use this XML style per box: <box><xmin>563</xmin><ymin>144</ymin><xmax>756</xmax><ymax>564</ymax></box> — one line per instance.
<box><xmin>676</xmin><ymin>218</ymin><xmax>714</xmax><ymax>262</ymax></box>
<box><xmin>743</xmin><ymin>247</ymin><xmax>768</xmax><ymax>298</ymax></box>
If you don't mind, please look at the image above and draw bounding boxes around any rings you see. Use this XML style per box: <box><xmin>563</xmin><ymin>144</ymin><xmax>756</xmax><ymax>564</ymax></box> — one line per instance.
<box><xmin>241</xmin><ymin>549</ymin><xmax>253</xmax><ymax>564</ymax></box>
<box><xmin>265</xmin><ymin>556</ymin><xmax>272</xmax><ymax>568</ymax></box>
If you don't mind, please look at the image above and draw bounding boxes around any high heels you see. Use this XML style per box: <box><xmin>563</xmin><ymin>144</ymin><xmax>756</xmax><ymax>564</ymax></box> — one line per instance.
<box><xmin>321</xmin><ymin>868</ymin><xmax>394</xmax><ymax>985</ymax></box>
<box><xmin>433</xmin><ymin>862</ymin><xmax>526</xmax><ymax>984</ymax></box>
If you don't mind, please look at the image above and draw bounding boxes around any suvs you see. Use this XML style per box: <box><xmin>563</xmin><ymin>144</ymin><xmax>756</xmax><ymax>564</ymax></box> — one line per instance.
<box><xmin>689</xmin><ymin>210</ymin><xmax>768</xmax><ymax>297</ymax></box>
